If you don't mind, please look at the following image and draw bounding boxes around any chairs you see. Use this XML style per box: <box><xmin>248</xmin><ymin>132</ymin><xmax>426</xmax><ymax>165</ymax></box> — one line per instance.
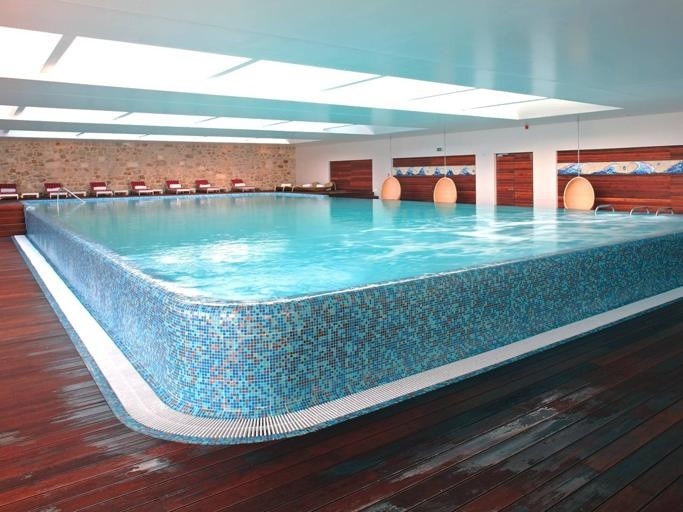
<box><xmin>0</xmin><ymin>179</ymin><xmax>255</xmax><ymax>201</ymax></box>
